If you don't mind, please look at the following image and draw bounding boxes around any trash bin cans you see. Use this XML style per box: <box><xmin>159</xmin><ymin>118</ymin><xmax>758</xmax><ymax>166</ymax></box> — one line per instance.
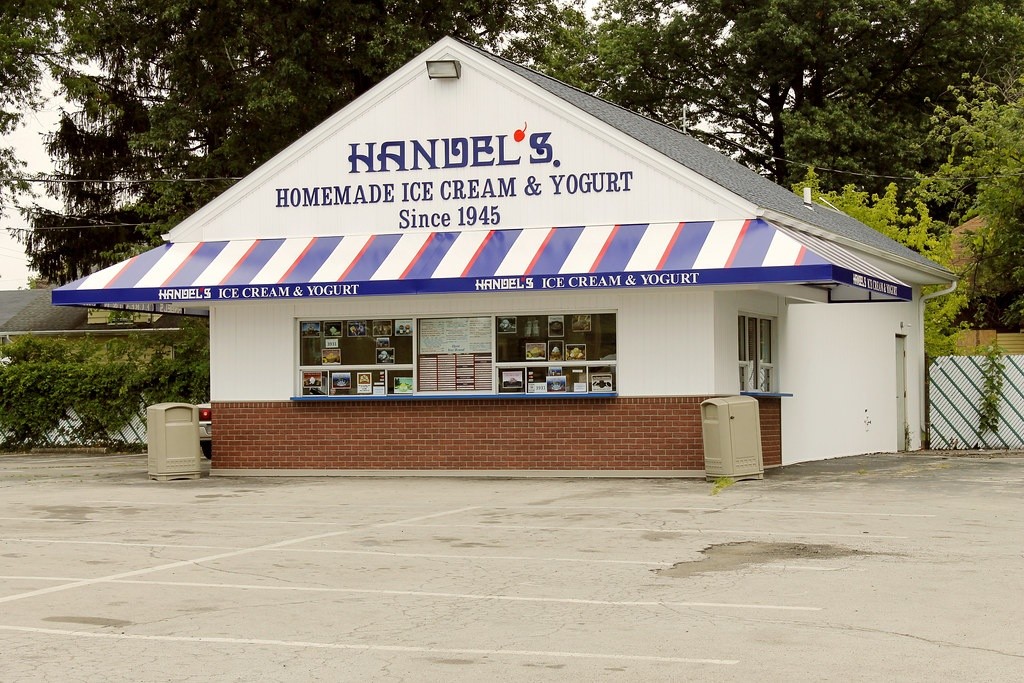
<box><xmin>699</xmin><ymin>394</ymin><xmax>764</xmax><ymax>481</ymax></box>
<box><xmin>146</xmin><ymin>402</ymin><xmax>201</xmax><ymax>482</ymax></box>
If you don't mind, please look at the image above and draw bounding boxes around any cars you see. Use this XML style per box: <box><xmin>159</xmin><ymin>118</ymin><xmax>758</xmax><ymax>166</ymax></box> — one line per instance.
<box><xmin>197</xmin><ymin>403</ymin><xmax>213</xmax><ymax>460</ymax></box>
